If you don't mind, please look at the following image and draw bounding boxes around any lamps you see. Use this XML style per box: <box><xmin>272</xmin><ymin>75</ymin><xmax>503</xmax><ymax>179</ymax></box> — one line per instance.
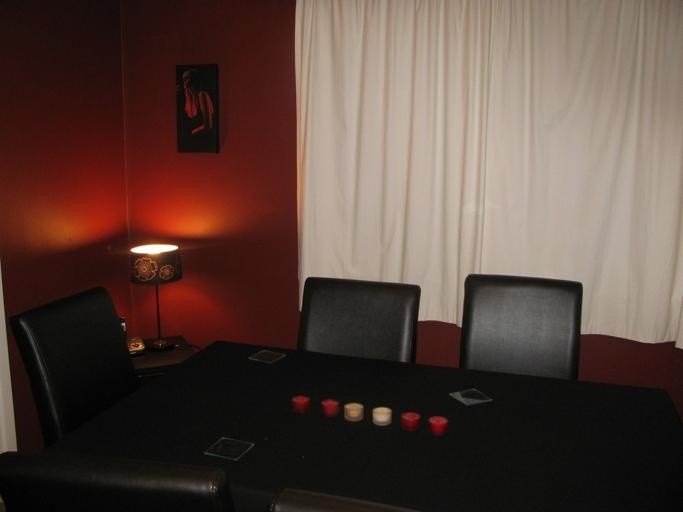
<box><xmin>129</xmin><ymin>243</ymin><xmax>182</xmax><ymax>349</ymax></box>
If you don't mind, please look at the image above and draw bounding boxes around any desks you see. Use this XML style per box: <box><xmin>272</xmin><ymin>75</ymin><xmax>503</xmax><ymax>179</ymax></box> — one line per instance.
<box><xmin>47</xmin><ymin>342</ymin><xmax>683</xmax><ymax>512</ymax></box>
